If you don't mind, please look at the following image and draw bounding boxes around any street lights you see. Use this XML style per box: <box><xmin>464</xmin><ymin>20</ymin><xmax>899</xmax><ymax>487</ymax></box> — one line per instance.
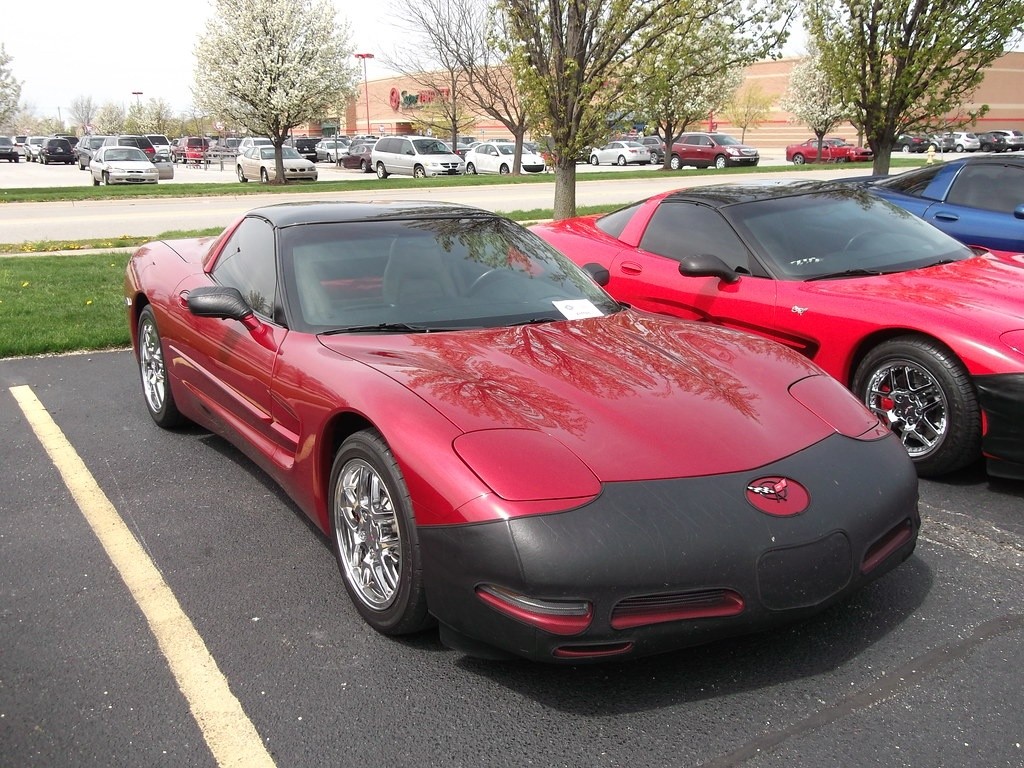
<box><xmin>354</xmin><ymin>53</ymin><xmax>374</xmax><ymax>135</ymax></box>
<box><xmin>132</xmin><ymin>91</ymin><xmax>143</xmax><ymax>105</ymax></box>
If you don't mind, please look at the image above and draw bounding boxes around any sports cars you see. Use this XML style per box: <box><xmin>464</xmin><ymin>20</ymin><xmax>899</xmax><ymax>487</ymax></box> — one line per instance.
<box><xmin>126</xmin><ymin>198</ymin><xmax>923</xmax><ymax>667</ymax></box>
<box><xmin>504</xmin><ymin>176</ymin><xmax>1024</xmax><ymax>519</ymax></box>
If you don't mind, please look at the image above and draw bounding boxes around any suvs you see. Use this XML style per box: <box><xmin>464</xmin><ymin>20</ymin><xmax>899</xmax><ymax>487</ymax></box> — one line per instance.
<box><xmin>10</xmin><ymin>135</ymin><xmax>28</xmax><ymax>156</ymax></box>
<box><xmin>0</xmin><ymin>136</ymin><xmax>20</xmax><ymax>163</ymax></box>
<box><xmin>23</xmin><ymin>136</ymin><xmax>48</xmax><ymax>162</ymax></box>
<box><xmin>37</xmin><ymin>136</ymin><xmax>76</xmax><ymax>165</ymax></box>
<box><xmin>370</xmin><ymin>134</ymin><xmax>466</xmax><ymax>179</ymax></box>
<box><xmin>662</xmin><ymin>132</ymin><xmax>760</xmax><ymax>171</ymax></box>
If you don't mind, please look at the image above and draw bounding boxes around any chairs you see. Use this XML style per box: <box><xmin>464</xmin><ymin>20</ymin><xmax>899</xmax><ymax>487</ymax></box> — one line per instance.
<box><xmin>385</xmin><ymin>235</ymin><xmax>458</xmax><ymax>311</ymax></box>
<box><xmin>504</xmin><ymin>149</ymin><xmax>509</xmax><ymax>153</ymax></box>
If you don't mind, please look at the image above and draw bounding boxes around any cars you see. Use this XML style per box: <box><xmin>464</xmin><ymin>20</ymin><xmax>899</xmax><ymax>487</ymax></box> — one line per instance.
<box><xmin>235</xmin><ymin>144</ymin><xmax>318</xmax><ymax>184</ymax></box>
<box><xmin>785</xmin><ymin>138</ymin><xmax>874</xmax><ymax>166</ymax></box>
<box><xmin>943</xmin><ymin>131</ymin><xmax>981</xmax><ymax>153</ymax></box>
<box><xmin>892</xmin><ymin>132</ymin><xmax>932</xmax><ymax>154</ymax></box>
<box><xmin>971</xmin><ymin>130</ymin><xmax>1006</xmax><ymax>154</ymax></box>
<box><xmin>589</xmin><ymin>141</ymin><xmax>651</xmax><ymax>168</ymax></box>
<box><xmin>825</xmin><ymin>151</ymin><xmax>1024</xmax><ymax>257</ymax></box>
<box><xmin>924</xmin><ymin>133</ymin><xmax>957</xmax><ymax>154</ymax></box>
<box><xmin>992</xmin><ymin>129</ymin><xmax>1024</xmax><ymax>152</ymax></box>
<box><xmin>89</xmin><ymin>145</ymin><xmax>174</xmax><ymax>186</ymax></box>
<box><xmin>50</xmin><ymin>130</ymin><xmax>670</xmax><ymax>174</ymax></box>
<box><xmin>464</xmin><ymin>141</ymin><xmax>548</xmax><ymax>176</ymax></box>
